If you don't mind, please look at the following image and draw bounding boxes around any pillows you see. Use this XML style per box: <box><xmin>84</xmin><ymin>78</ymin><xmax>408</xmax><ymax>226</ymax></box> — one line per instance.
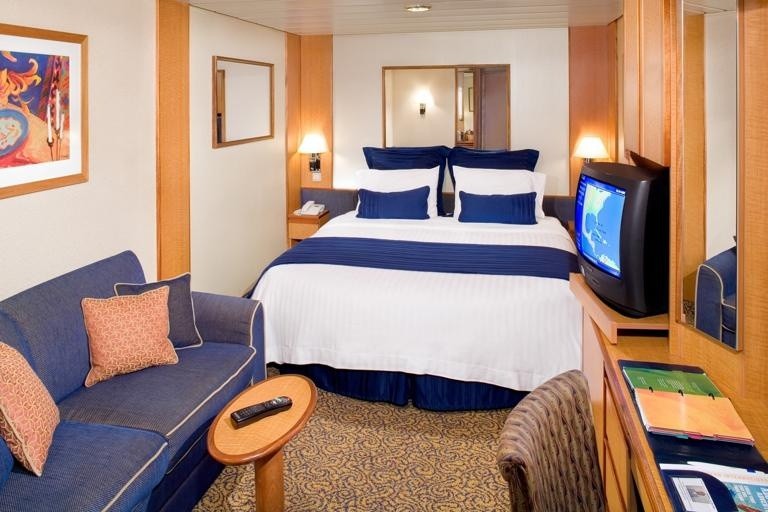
<box><xmin>355</xmin><ymin>146</ymin><xmax>547</xmax><ymax>224</ymax></box>
<box><xmin>81</xmin><ymin>286</ymin><xmax>179</xmax><ymax>387</ymax></box>
<box><xmin>115</xmin><ymin>273</ymin><xmax>203</xmax><ymax>351</ymax></box>
<box><xmin>0</xmin><ymin>341</ymin><xmax>61</xmax><ymax>477</ymax></box>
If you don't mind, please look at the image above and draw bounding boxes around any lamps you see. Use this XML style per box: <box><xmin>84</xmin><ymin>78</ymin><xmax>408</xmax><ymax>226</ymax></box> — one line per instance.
<box><xmin>419</xmin><ymin>102</ymin><xmax>426</xmax><ymax>115</ymax></box>
<box><xmin>573</xmin><ymin>135</ymin><xmax>609</xmax><ymax>165</ymax></box>
<box><xmin>295</xmin><ymin>134</ymin><xmax>329</xmax><ymax>172</ymax></box>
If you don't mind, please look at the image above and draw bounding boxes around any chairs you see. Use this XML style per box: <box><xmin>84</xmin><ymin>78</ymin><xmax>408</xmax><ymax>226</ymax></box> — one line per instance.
<box><xmin>496</xmin><ymin>369</ymin><xmax>609</xmax><ymax>512</ymax></box>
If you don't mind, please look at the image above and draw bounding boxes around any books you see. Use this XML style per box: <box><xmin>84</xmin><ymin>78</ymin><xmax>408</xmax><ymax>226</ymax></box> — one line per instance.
<box><xmin>622</xmin><ymin>366</ymin><xmax>723</xmax><ymax>397</ymax></box>
<box><xmin>634</xmin><ymin>387</ymin><xmax>756</xmax><ymax>447</ymax></box>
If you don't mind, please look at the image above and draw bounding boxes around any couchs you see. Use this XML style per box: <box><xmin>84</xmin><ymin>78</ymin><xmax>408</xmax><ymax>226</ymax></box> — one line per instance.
<box><xmin>0</xmin><ymin>250</ymin><xmax>267</xmax><ymax>512</ymax></box>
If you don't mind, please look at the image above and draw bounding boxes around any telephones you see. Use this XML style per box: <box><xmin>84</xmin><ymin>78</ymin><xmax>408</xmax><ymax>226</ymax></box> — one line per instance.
<box><xmin>293</xmin><ymin>200</ymin><xmax>326</xmax><ymax>216</ymax></box>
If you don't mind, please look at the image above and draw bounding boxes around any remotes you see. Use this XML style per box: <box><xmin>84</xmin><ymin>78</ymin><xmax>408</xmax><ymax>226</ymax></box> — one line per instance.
<box><xmin>232</xmin><ymin>395</ymin><xmax>292</xmax><ymax>428</ymax></box>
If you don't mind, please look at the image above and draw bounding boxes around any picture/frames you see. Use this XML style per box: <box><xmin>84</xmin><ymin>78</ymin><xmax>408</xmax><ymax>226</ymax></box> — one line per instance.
<box><xmin>468</xmin><ymin>87</ymin><xmax>474</xmax><ymax>112</ymax></box>
<box><xmin>1</xmin><ymin>22</ymin><xmax>89</xmax><ymax>201</ymax></box>
<box><xmin>211</xmin><ymin>56</ymin><xmax>274</xmax><ymax>149</ymax></box>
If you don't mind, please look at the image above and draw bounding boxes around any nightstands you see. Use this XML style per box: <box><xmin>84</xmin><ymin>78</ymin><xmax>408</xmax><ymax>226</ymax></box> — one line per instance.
<box><xmin>287</xmin><ymin>211</ymin><xmax>330</xmax><ymax>249</ymax></box>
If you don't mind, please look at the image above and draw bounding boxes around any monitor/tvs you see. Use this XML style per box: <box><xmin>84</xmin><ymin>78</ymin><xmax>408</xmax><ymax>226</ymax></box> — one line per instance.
<box><xmin>569</xmin><ymin>162</ymin><xmax>669</xmax><ymax>318</ymax></box>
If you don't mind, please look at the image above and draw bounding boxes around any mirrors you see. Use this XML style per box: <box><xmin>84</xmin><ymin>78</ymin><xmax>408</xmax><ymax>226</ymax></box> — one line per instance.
<box><xmin>382</xmin><ymin>64</ymin><xmax>511</xmax><ymax>151</ymax></box>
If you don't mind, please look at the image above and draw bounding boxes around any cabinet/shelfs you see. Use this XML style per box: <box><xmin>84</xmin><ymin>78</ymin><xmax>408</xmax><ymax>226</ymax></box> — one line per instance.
<box><xmin>570</xmin><ymin>0</ymin><xmax>768</xmax><ymax>512</ymax></box>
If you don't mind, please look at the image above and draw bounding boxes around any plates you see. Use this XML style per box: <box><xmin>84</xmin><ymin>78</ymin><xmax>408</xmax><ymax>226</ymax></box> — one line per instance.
<box><xmin>0</xmin><ymin>108</ymin><xmax>30</xmax><ymax>156</ymax></box>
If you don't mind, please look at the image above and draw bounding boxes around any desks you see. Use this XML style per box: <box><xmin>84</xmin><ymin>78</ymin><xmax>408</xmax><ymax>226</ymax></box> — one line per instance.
<box><xmin>207</xmin><ymin>374</ymin><xmax>317</xmax><ymax>512</ymax></box>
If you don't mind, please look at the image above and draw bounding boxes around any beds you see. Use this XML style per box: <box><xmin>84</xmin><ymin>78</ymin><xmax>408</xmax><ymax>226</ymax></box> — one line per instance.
<box><xmin>249</xmin><ymin>190</ymin><xmax>583</xmax><ymax>412</ymax></box>
